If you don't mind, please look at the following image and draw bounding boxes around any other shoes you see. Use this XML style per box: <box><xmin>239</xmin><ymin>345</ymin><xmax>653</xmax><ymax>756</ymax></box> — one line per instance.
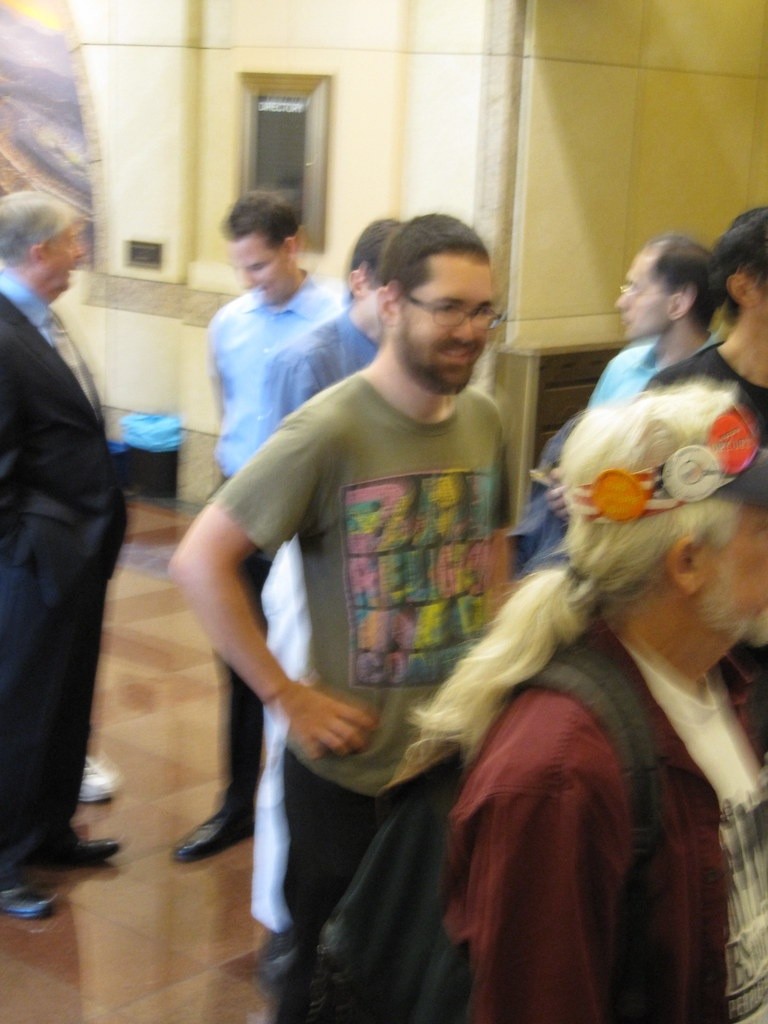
<box><xmin>78</xmin><ymin>752</ymin><xmax>119</xmax><ymax>804</ymax></box>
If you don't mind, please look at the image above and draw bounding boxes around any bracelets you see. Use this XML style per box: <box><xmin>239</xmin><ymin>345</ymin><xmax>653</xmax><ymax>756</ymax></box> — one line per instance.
<box><xmin>260</xmin><ymin>679</ymin><xmax>297</xmax><ymax>705</ymax></box>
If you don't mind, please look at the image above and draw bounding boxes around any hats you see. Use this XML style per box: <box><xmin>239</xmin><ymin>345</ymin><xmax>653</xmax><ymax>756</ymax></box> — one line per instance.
<box><xmin>570</xmin><ymin>402</ymin><xmax>768</xmax><ymax>528</ymax></box>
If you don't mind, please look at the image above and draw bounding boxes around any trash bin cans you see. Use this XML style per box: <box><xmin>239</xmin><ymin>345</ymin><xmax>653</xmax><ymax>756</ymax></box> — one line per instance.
<box><xmin>120</xmin><ymin>412</ymin><xmax>180</xmax><ymax>498</ymax></box>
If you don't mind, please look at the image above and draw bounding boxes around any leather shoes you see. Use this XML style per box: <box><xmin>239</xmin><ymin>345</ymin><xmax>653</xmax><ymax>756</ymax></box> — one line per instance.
<box><xmin>33</xmin><ymin>841</ymin><xmax>119</xmax><ymax>867</ymax></box>
<box><xmin>174</xmin><ymin>808</ymin><xmax>255</xmax><ymax>864</ymax></box>
<box><xmin>0</xmin><ymin>881</ymin><xmax>59</xmax><ymax>922</ymax></box>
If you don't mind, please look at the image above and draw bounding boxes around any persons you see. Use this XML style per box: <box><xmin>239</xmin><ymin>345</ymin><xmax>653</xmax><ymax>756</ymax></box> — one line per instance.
<box><xmin>166</xmin><ymin>192</ymin><xmax>513</xmax><ymax>1024</ymax></box>
<box><xmin>0</xmin><ymin>186</ymin><xmax>127</xmax><ymax>919</ymax></box>
<box><xmin>585</xmin><ymin>207</ymin><xmax>768</xmax><ymax>444</ymax></box>
<box><xmin>408</xmin><ymin>379</ymin><xmax>768</xmax><ymax>1024</ymax></box>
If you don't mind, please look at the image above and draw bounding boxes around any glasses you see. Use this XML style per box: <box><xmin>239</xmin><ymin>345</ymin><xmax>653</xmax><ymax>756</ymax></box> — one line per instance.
<box><xmin>401</xmin><ymin>292</ymin><xmax>505</xmax><ymax>331</ymax></box>
<box><xmin>620</xmin><ymin>282</ymin><xmax>645</xmax><ymax>299</ymax></box>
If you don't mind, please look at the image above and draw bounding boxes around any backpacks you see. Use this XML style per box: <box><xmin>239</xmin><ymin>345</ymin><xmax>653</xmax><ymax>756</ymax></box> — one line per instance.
<box><xmin>307</xmin><ymin>644</ymin><xmax>663</xmax><ymax>1024</ymax></box>
